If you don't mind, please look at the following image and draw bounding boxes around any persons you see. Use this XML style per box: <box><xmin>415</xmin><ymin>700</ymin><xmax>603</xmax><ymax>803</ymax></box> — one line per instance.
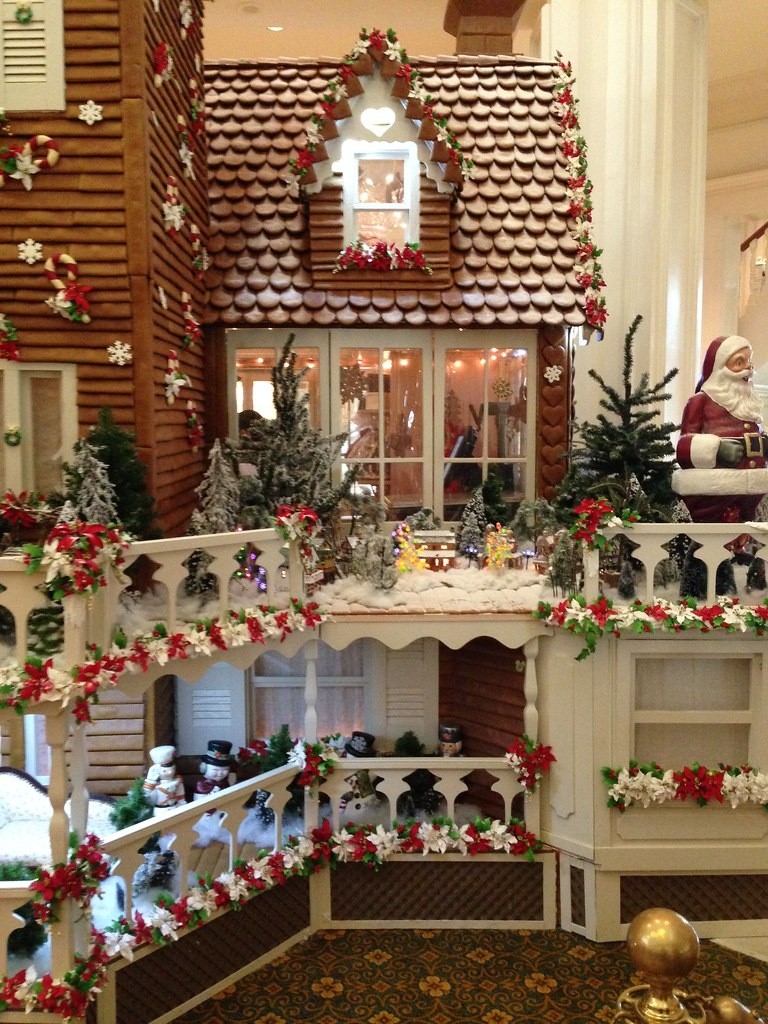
<box><xmin>676</xmin><ymin>335</ymin><xmax>768</xmax><ymax>565</ymax></box>
<box><xmin>142</xmin><ymin>745</ymin><xmax>187</xmax><ymax>817</ymax></box>
<box><xmin>432</xmin><ymin>724</ymin><xmax>467</xmax><ymax>803</ymax></box>
<box><xmin>196</xmin><ymin>739</ymin><xmax>238</xmax><ymax>814</ymax></box>
